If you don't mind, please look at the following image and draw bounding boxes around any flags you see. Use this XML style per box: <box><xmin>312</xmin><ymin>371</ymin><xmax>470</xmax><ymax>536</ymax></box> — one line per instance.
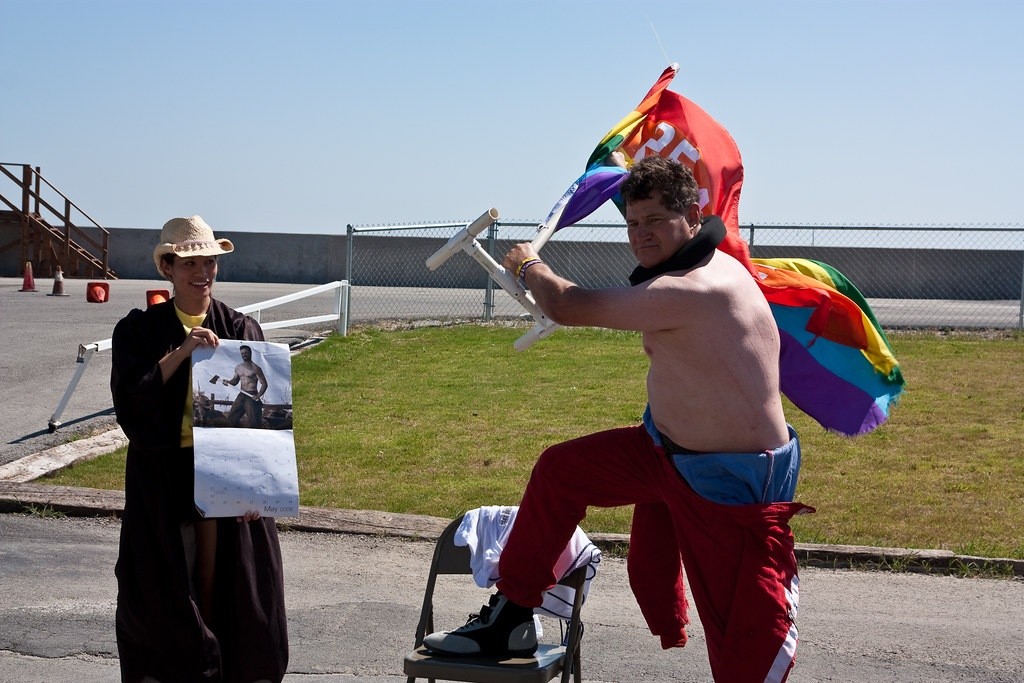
<box><xmin>536</xmin><ymin>61</ymin><xmax>909</xmax><ymax>437</ymax></box>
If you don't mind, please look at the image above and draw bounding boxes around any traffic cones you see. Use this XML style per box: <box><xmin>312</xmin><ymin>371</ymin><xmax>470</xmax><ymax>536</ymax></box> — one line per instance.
<box><xmin>146</xmin><ymin>288</ymin><xmax>170</xmax><ymax>312</ymax></box>
<box><xmin>46</xmin><ymin>266</ymin><xmax>70</xmax><ymax>298</ymax></box>
<box><xmin>18</xmin><ymin>262</ymin><xmax>40</xmax><ymax>293</ymax></box>
<box><xmin>86</xmin><ymin>282</ymin><xmax>110</xmax><ymax>303</ymax></box>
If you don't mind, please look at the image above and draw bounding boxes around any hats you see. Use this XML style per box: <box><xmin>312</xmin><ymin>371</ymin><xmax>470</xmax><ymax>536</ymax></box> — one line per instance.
<box><xmin>154</xmin><ymin>214</ymin><xmax>234</xmax><ymax>283</ymax></box>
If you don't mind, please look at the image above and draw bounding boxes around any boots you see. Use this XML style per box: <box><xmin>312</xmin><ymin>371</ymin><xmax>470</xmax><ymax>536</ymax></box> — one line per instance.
<box><xmin>424</xmin><ymin>592</ymin><xmax>537</xmax><ymax>659</ymax></box>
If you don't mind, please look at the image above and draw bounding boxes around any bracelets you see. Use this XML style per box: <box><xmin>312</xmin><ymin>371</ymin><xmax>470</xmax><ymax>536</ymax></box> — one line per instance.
<box><xmin>519</xmin><ymin>260</ymin><xmax>542</xmax><ymax>284</ymax></box>
<box><xmin>514</xmin><ymin>256</ymin><xmax>541</xmax><ymax>280</ymax></box>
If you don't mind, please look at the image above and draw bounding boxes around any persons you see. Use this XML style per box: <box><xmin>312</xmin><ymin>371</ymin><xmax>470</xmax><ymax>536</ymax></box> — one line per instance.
<box><xmin>421</xmin><ymin>155</ymin><xmax>818</xmax><ymax>683</ymax></box>
<box><xmin>221</xmin><ymin>345</ymin><xmax>268</xmax><ymax>430</ymax></box>
<box><xmin>109</xmin><ymin>212</ymin><xmax>290</xmax><ymax>683</ymax></box>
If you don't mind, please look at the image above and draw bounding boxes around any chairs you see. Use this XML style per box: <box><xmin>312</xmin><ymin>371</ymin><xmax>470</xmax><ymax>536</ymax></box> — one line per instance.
<box><xmin>403</xmin><ymin>503</ymin><xmax>601</xmax><ymax>683</ymax></box>
